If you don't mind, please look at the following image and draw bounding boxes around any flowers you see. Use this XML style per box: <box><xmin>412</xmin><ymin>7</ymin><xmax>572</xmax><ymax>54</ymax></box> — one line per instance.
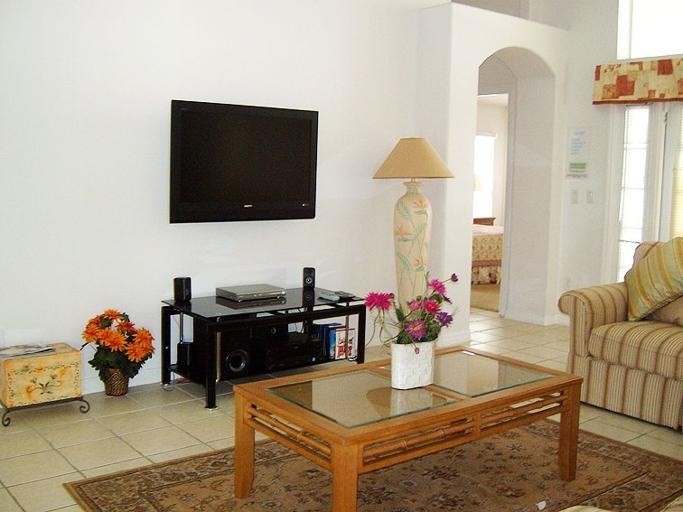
<box><xmin>365</xmin><ymin>273</ymin><xmax>458</xmax><ymax>345</ymax></box>
<box><xmin>79</xmin><ymin>310</ymin><xmax>155</xmax><ymax>379</ymax></box>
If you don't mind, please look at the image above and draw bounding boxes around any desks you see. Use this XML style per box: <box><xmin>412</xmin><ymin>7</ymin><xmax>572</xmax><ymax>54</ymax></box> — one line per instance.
<box><xmin>161</xmin><ymin>287</ymin><xmax>366</xmax><ymax>410</ymax></box>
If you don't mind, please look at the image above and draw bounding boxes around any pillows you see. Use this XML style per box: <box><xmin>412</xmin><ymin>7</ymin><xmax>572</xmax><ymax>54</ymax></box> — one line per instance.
<box><xmin>625</xmin><ymin>237</ymin><xmax>683</xmax><ymax>321</ymax></box>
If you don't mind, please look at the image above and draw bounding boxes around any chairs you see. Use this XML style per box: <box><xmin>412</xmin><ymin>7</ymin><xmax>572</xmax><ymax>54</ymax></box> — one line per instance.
<box><xmin>558</xmin><ymin>241</ymin><xmax>683</xmax><ymax>430</ymax></box>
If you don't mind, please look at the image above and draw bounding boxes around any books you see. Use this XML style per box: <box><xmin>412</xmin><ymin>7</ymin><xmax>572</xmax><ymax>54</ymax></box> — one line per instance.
<box><xmin>312</xmin><ymin>323</ymin><xmax>357</xmax><ymax>360</ymax></box>
<box><xmin>319</xmin><ymin>290</ymin><xmax>356</xmax><ymax>301</ymax></box>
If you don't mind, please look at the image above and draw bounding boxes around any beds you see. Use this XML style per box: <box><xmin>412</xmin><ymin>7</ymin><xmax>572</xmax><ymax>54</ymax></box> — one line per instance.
<box><xmin>472</xmin><ymin>224</ymin><xmax>503</xmax><ymax>285</ymax></box>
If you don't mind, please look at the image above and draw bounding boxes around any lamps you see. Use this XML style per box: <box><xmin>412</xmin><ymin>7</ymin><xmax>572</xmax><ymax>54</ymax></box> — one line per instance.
<box><xmin>373</xmin><ymin>138</ymin><xmax>454</xmax><ymax>355</ymax></box>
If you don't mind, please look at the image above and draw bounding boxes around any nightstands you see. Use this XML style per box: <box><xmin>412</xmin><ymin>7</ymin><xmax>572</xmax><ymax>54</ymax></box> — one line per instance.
<box><xmin>473</xmin><ymin>218</ymin><xmax>495</xmax><ymax>225</ymax></box>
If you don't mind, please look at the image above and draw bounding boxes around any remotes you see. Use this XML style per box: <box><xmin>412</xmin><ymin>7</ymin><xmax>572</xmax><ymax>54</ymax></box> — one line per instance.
<box><xmin>320</xmin><ymin>291</ymin><xmax>340</xmax><ymax>302</ymax></box>
<box><xmin>335</xmin><ymin>290</ymin><xmax>355</xmax><ymax>299</ymax></box>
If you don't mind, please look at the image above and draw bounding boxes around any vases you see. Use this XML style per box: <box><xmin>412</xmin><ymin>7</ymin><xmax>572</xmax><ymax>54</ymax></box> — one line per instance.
<box><xmin>391</xmin><ymin>341</ymin><xmax>436</xmax><ymax>389</ymax></box>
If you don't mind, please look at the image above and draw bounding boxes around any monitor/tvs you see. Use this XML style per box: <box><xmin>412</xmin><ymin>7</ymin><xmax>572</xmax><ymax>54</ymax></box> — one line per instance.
<box><xmin>169</xmin><ymin>98</ymin><xmax>318</xmax><ymax>221</ymax></box>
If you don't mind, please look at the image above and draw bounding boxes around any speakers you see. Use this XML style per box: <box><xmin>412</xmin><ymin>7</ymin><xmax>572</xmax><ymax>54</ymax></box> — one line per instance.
<box><xmin>175</xmin><ymin>277</ymin><xmax>190</xmax><ymax>302</ymax></box>
<box><xmin>303</xmin><ymin>267</ymin><xmax>315</xmax><ymax>288</ymax></box>
<box><xmin>194</xmin><ymin>317</ymin><xmax>253</xmax><ymax>375</ymax></box>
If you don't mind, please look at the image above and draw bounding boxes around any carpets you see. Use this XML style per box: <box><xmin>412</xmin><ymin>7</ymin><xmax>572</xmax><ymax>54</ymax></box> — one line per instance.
<box><xmin>64</xmin><ymin>408</ymin><xmax>683</xmax><ymax>511</ymax></box>
